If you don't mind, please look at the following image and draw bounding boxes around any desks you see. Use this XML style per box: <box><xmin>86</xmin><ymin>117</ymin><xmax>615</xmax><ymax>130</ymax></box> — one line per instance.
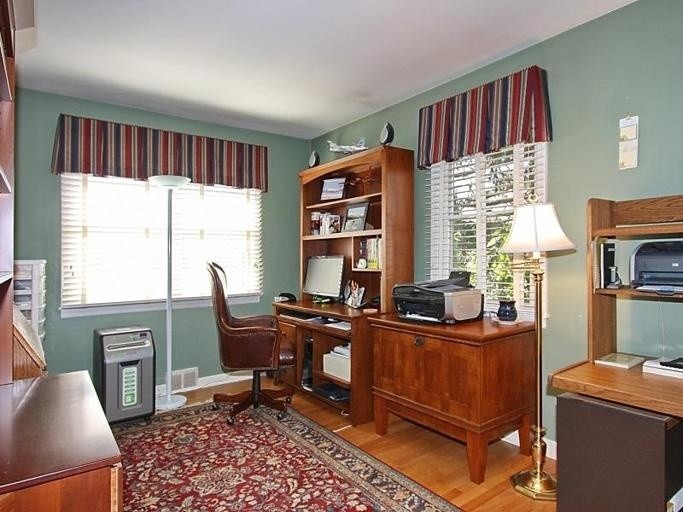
<box><xmin>268</xmin><ymin>296</ymin><xmax>381</xmax><ymax>428</ymax></box>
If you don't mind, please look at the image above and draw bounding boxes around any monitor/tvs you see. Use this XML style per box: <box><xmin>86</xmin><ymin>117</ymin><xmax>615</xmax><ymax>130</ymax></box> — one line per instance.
<box><xmin>303</xmin><ymin>255</ymin><xmax>346</xmax><ymax>303</ymax></box>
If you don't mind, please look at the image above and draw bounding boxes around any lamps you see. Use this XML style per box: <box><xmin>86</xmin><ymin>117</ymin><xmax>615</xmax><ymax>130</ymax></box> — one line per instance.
<box><xmin>495</xmin><ymin>199</ymin><xmax>576</xmax><ymax>504</ymax></box>
<box><xmin>143</xmin><ymin>172</ymin><xmax>192</xmax><ymax>411</ymax></box>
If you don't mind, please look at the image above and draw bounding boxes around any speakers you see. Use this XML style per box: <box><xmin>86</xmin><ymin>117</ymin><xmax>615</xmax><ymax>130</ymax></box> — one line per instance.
<box><xmin>380</xmin><ymin>121</ymin><xmax>395</xmax><ymax>145</ymax></box>
<box><xmin>308</xmin><ymin>150</ymin><xmax>320</xmax><ymax>167</ymax></box>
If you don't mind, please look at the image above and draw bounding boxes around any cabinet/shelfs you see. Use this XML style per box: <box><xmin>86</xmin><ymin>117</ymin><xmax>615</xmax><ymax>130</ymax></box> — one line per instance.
<box><xmin>0</xmin><ymin>368</ymin><xmax>123</xmax><ymax>510</ymax></box>
<box><xmin>8</xmin><ymin>257</ymin><xmax>48</xmax><ymax>345</ymax></box>
<box><xmin>295</xmin><ymin>144</ymin><xmax>416</xmax><ymax>311</ymax></box>
<box><xmin>0</xmin><ymin>0</ymin><xmax>18</xmax><ymax>387</ymax></box>
<box><xmin>366</xmin><ymin>307</ymin><xmax>539</xmax><ymax>485</ymax></box>
<box><xmin>544</xmin><ymin>192</ymin><xmax>682</xmax><ymax>511</ymax></box>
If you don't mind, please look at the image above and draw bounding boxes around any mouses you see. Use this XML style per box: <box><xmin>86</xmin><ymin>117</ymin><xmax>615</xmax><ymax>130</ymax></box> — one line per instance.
<box><xmin>327</xmin><ymin>318</ymin><xmax>337</xmax><ymax>323</ymax></box>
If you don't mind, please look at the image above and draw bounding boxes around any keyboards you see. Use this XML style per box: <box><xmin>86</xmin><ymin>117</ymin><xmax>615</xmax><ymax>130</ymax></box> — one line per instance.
<box><xmin>280</xmin><ymin>310</ymin><xmax>318</xmax><ymax>322</ymax></box>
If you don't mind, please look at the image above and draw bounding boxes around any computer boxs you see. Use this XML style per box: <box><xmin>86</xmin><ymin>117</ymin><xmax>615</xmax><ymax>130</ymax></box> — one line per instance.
<box><xmin>301</xmin><ymin>337</ymin><xmax>313</xmax><ymax>392</ymax></box>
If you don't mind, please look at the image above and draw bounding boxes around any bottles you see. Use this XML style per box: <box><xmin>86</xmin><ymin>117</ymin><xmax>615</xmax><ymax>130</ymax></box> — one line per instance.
<box><xmin>497</xmin><ymin>299</ymin><xmax>517</xmax><ymax>322</ymax></box>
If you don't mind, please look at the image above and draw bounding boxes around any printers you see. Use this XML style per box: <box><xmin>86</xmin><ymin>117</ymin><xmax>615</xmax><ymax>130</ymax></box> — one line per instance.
<box><xmin>392</xmin><ymin>271</ymin><xmax>484</xmax><ymax>325</ymax></box>
<box><xmin>629</xmin><ymin>239</ymin><xmax>683</xmax><ymax>294</ymax></box>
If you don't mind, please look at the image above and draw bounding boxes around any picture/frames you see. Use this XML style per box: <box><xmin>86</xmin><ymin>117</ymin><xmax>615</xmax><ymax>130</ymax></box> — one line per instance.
<box><xmin>315</xmin><ymin>175</ymin><xmax>349</xmax><ymax>203</ymax></box>
<box><xmin>338</xmin><ymin>203</ymin><xmax>370</xmax><ymax>233</ymax></box>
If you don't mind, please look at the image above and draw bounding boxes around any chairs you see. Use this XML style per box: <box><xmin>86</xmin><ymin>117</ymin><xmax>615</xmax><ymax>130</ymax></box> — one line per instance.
<box><xmin>205</xmin><ymin>260</ymin><xmax>295</xmax><ymax>425</ymax></box>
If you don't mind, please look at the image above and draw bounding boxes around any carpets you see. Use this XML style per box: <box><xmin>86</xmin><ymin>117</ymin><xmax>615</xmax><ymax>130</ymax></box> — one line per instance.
<box><xmin>108</xmin><ymin>388</ymin><xmax>472</xmax><ymax>511</ymax></box>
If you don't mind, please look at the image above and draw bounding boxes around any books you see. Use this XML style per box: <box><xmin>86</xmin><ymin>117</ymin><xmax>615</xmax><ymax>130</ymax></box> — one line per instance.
<box><xmin>309</xmin><ymin>212</ymin><xmax>339</xmax><ymax>234</ymax></box>
<box><xmin>328</xmin><ymin>343</ymin><xmax>351</xmax><ymax>358</ymax></box>
<box><xmin>360</xmin><ymin>237</ymin><xmax>381</xmax><ymax>269</ymax></box>
<box><xmin>642</xmin><ymin>357</ymin><xmax>683</xmax><ymax>380</ymax></box>
<box><xmin>595</xmin><ymin>352</ymin><xmax>645</xmax><ymax>369</ymax></box>
